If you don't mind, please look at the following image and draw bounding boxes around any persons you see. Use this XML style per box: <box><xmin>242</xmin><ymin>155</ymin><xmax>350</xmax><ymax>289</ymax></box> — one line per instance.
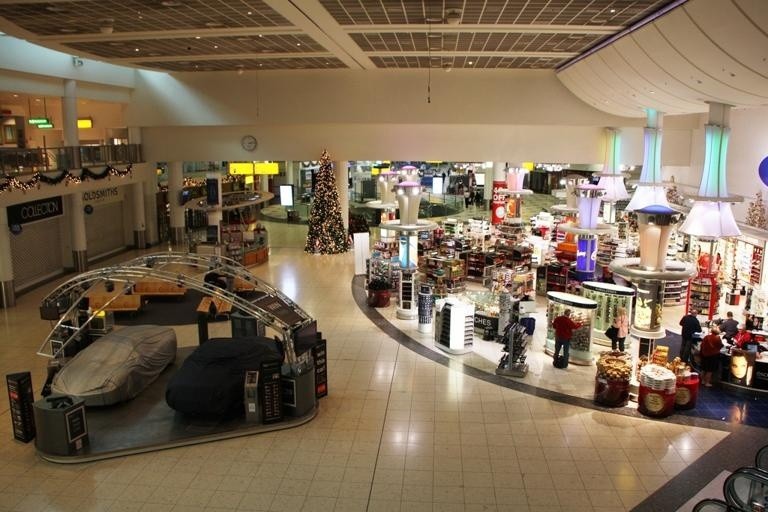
<box><xmin>717</xmin><ymin>252</ymin><xmax>722</xmax><ymax>270</ymax></box>
<box><xmin>552</xmin><ymin>309</ymin><xmax>583</xmax><ymax>362</ymax></box>
<box><xmin>730</xmin><ymin>349</ymin><xmax>748</xmax><ymax>379</ymax></box>
<box><xmin>730</xmin><ymin>323</ymin><xmax>751</xmax><ymax>348</ymax></box>
<box><xmin>742</xmin><ymin>334</ymin><xmax>763</xmax><ymax>386</ymax></box>
<box><xmin>611</xmin><ymin>307</ymin><xmax>629</xmax><ymax>352</ymax></box>
<box><xmin>679</xmin><ymin>309</ymin><xmax>702</xmax><ymax>363</ymax></box>
<box><xmin>731</xmin><ymin>269</ymin><xmax>738</xmax><ymax>293</ymax></box>
<box><xmin>739</xmin><ymin>305</ymin><xmax>751</xmax><ymax>329</ymax></box>
<box><xmin>698</xmin><ymin>245</ymin><xmax>701</xmax><ymax>255</ymax></box>
<box><xmin>717</xmin><ymin>311</ymin><xmax>739</xmax><ymax>345</ymax></box>
<box><xmin>699</xmin><ymin>328</ymin><xmax>724</xmax><ymax>387</ymax></box>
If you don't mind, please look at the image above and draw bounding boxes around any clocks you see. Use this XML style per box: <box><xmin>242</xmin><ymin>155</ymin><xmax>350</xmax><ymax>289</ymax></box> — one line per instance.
<box><xmin>241</xmin><ymin>135</ymin><xmax>258</xmax><ymax>152</ymax></box>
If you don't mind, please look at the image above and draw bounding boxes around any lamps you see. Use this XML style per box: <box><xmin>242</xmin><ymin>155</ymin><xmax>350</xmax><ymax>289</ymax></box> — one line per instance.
<box><xmin>441</xmin><ymin>63</ymin><xmax>453</xmax><ymax>73</ymax></box>
<box><xmin>98</xmin><ymin>18</ymin><xmax>115</xmax><ymax>35</ymax></box>
<box><xmin>446</xmin><ymin>8</ymin><xmax>463</xmax><ymax>26</ymax></box>
<box><xmin>233</xmin><ymin>65</ymin><xmax>244</xmax><ymax>76</ymax></box>
<box><xmin>591</xmin><ymin>100</ymin><xmax>747</xmax><ymax>240</ymax></box>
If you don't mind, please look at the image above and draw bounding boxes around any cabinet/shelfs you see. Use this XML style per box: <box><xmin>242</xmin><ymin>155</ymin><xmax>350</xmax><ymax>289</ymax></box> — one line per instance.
<box><xmin>684</xmin><ymin>272</ymin><xmax>719</xmax><ymax>321</ymax></box>
<box><xmin>365</xmin><ymin>237</ymin><xmax>572</xmax><ymax>355</ymax></box>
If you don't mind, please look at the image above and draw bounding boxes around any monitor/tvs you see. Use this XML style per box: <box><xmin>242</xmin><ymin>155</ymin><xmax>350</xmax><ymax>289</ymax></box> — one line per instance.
<box><xmin>748</xmin><ymin>317</ymin><xmax>763</xmax><ymax>330</ymax></box>
<box><xmin>242</xmin><ymin>231</ymin><xmax>255</xmax><ymax>241</ymax></box>
<box><xmin>745</xmin><ymin>343</ymin><xmax>759</xmax><ymax>353</ymax></box>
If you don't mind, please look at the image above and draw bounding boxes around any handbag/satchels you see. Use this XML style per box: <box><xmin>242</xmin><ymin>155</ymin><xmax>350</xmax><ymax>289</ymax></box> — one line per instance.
<box><xmin>605</xmin><ymin>324</ymin><xmax>619</xmax><ymax>340</ymax></box>
